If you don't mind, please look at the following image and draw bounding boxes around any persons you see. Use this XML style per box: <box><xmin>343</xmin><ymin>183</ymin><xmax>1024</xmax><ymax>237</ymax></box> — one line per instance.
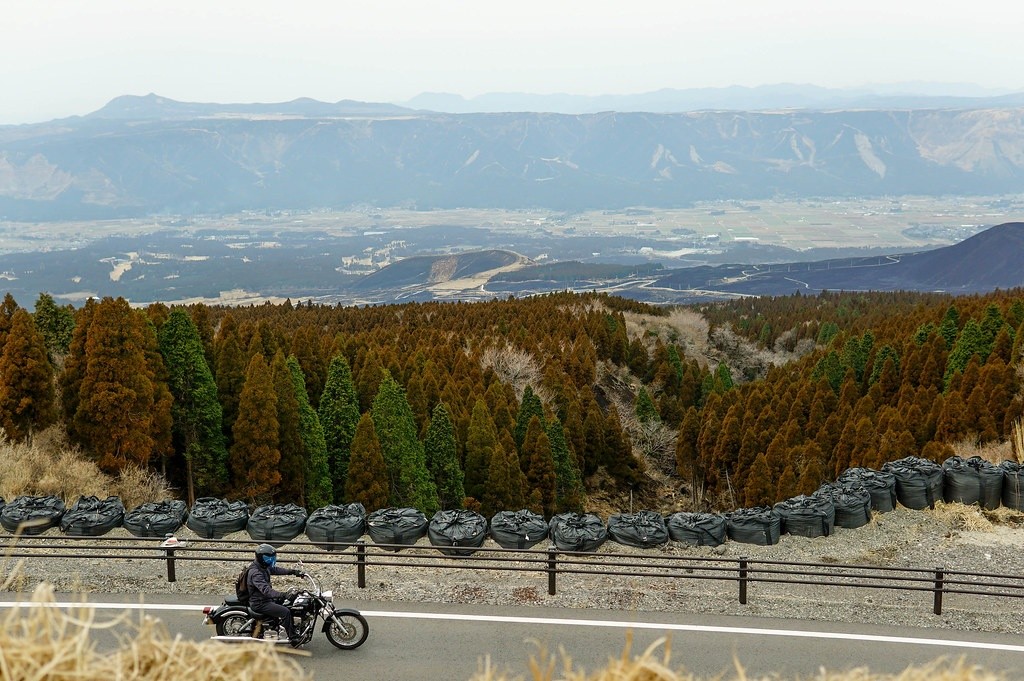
<box><xmin>249</xmin><ymin>544</ymin><xmax>307</xmax><ymax>649</ymax></box>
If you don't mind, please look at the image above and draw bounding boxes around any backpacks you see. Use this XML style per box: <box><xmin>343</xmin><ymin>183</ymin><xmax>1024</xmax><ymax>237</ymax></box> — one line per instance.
<box><xmin>236</xmin><ymin>564</ymin><xmax>269</xmax><ymax>602</ymax></box>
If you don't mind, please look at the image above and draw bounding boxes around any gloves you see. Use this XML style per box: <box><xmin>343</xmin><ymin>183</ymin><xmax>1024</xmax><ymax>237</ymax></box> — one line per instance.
<box><xmin>282</xmin><ymin>592</ymin><xmax>294</xmax><ymax>601</ymax></box>
<box><xmin>293</xmin><ymin>569</ymin><xmax>302</xmax><ymax>578</ymax></box>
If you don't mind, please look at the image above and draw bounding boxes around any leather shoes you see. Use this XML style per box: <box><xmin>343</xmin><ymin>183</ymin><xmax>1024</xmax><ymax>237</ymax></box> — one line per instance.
<box><xmin>290</xmin><ymin>634</ymin><xmax>308</xmax><ymax>649</ymax></box>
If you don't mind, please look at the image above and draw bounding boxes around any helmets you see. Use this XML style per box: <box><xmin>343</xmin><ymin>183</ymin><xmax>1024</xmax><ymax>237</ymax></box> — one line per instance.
<box><xmin>255</xmin><ymin>544</ymin><xmax>277</xmax><ymax>570</ymax></box>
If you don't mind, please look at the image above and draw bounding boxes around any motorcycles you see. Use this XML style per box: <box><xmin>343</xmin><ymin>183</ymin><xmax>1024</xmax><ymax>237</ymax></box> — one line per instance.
<box><xmin>202</xmin><ymin>554</ymin><xmax>370</xmax><ymax>650</ymax></box>
